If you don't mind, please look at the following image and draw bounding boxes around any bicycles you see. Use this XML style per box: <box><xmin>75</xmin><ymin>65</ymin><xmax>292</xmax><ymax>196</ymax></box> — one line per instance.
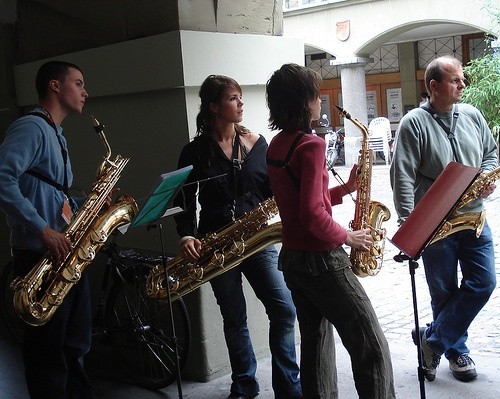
<box><xmin>85</xmin><ymin>226</ymin><xmax>192</xmax><ymax>393</ymax></box>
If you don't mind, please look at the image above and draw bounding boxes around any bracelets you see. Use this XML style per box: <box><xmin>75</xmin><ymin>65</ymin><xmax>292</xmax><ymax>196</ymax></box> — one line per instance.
<box><xmin>179</xmin><ymin>236</ymin><xmax>195</xmax><ymax>246</ymax></box>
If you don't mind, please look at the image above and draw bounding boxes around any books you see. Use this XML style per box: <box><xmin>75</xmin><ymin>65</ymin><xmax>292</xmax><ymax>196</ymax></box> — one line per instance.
<box><xmin>129</xmin><ymin>165</ymin><xmax>193</xmax><ymax>227</ymax></box>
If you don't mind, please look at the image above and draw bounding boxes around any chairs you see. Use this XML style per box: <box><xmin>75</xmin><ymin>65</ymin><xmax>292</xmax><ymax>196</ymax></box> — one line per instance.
<box><xmin>368</xmin><ymin>117</ymin><xmax>392</xmax><ymax>166</ymax></box>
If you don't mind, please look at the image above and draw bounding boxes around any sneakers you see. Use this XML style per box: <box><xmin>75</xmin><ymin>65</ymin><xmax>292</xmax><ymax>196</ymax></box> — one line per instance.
<box><xmin>449</xmin><ymin>355</ymin><xmax>478</xmax><ymax>382</ymax></box>
<box><xmin>411</xmin><ymin>327</ymin><xmax>442</xmax><ymax>382</ymax></box>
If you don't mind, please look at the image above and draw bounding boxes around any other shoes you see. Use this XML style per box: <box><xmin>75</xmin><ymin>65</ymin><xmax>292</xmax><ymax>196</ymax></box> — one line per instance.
<box><xmin>228</xmin><ymin>388</ymin><xmax>260</xmax><ymax>399</ymax></box>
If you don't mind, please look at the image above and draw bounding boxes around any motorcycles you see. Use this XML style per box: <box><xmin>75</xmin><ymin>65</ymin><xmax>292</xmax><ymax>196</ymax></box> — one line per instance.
<box><xmin>320</xmin><ymin>113</ymin><xmax>345</xmax><ymax>171</ymax></box>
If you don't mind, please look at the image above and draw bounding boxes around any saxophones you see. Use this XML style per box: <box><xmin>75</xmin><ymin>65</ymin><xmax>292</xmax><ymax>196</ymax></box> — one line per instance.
<box><xmin>426</xmin><ymin>165</ymin><xmax>500</xmax><ymax>246</ymax></box>
<box><xmin>331</xmin><ymin>102</ymin><xmax>391</xmax><ymax>278</ymax></box>
<box><xmin>143</xmin><ymin>193</ymin><xmax>285</xmax><ymax>304</ymax></box>
<box><xmin>9</xmin><ymin>112</ymin><xmax>139</xmax><ymax>328</ymax></box>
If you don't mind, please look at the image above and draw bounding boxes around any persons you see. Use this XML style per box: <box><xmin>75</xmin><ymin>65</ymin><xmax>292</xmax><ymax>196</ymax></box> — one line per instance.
<box><xmin>266</xmin><ymin>63</ymin><xmax>396</xmax><ymax>399</ymax></box>
<box><xmin>172</xmin><ymin>75</ymin><xmax>303</xmax><ymax>399</ymax></box>
<box><xmin>389</xmin><ymin>56</ymin><xmax>500</xmax><ymax>382</ymax></box>
<box><xmin>0</xmin><ymin>61</ymin><xmax>113</xmax><ymax>399</ymax></box>
